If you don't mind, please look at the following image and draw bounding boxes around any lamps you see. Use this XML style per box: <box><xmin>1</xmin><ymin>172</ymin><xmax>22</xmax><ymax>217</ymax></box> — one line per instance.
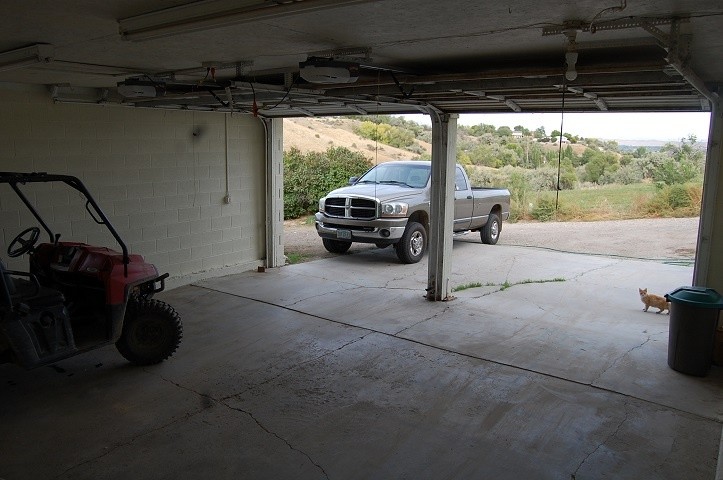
<box><xmin>0</xmin><ymin>0</ymin><xmax>363</xmax><ymax>106</ymax></box>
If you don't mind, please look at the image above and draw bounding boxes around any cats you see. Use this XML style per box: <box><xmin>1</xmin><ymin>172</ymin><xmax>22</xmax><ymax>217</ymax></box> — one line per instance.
<box><xmin>639</xmin><ymin>288</ymin><xmax>670</xmax><ymax>314</ymax></box>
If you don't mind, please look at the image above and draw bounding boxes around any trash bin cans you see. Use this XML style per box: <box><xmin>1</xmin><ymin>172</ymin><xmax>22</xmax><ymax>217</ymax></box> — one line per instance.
<box><xmin>665</xmin><ymin>286</ymin><xmax>723</xmax><ymax>378</ymax></box>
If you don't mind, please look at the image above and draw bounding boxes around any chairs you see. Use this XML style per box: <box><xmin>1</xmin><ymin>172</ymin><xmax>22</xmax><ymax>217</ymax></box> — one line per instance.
<box><xmin>0</xmin><ymin>262</ymin><xmax>53</xmax><ymax>306</ymax></box>
<box><xmin>409</xmin><ymin>176</ymin><xmax>420</xmax><ymax>181</ymax></box>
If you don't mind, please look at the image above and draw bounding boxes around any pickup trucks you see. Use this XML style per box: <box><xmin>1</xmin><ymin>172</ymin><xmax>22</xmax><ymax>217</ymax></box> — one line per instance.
<box><xmin>315</xmin><ymin>160</ymin><xmax>511</xmax><ymax>265</ymax></box>
